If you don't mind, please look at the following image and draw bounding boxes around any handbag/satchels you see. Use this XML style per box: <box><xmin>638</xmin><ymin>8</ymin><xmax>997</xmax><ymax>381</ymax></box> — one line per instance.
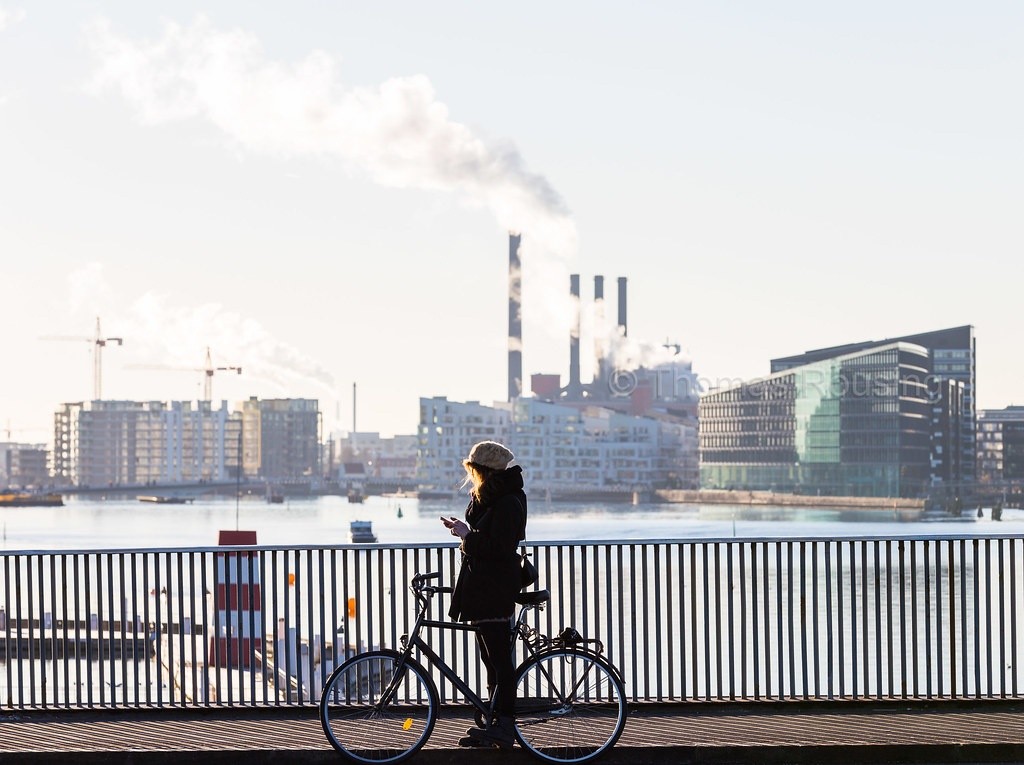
<box><xmin>521</xmin><ymin>553</ymin><xmax>539</xmax><ymax>589</ymax></box>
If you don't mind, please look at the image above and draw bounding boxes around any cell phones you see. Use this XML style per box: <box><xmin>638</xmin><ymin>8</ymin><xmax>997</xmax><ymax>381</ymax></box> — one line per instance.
<box><xmin>440</xmin><ymin>516</ymin><xmax>450</xmax><ymax>523</ymax></box>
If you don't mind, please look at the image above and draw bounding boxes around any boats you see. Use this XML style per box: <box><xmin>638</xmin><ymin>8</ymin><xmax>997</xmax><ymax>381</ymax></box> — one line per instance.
<box><xmin>397</xmin><ymin>507</ymin><xmax>403</xmax><ymax>518</ymax></box>
<box><xmin>136</xmin><ymin>495</ymin><xmax>195</xmax><ymax>503</ymax></box>
<box><xmin>348</xmin><ymin>482</ymin><xmax>363</xmax><ymax>503</ymax></box>
<box><xmin>266</xmin><ymin>484</ymin><xmax>284</xmax><ymax>502</ymax></box>
<box><xmin>416</xmin><ymin>482</ymin><xmax>458</xmax><ymax>497</ymax></box>
<box><xmin>0</xmin><ymin>631</ymin><xmax>155</xmax><ymax>648</ymax></box>
<box><xmin>0</xmin><ymin>488</ymin><xmax>63</xmax><ymax>505</ymax></box>
<box><xmin>350</xmin><ymin>520</ymin><xmax>378</xmax><ymax>543</ymax></box>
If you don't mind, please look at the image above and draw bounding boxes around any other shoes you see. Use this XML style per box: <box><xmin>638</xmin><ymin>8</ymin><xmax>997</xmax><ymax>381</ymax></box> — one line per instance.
<box><xmin>467</xmin><ymin>715</ymin><xmax>515</xmax><ymax>749</ymax></box>
<box><xmin>458</xmin><ymin>737</ymin><xmax>495</xmax><ymax>747</ymax></box>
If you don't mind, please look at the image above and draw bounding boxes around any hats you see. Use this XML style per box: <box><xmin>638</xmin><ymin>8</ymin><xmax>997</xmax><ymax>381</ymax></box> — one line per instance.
<box><xmin>468</xmin><ymin>440</ymin><xmax>515</xmax><ymax>470</ymax></box>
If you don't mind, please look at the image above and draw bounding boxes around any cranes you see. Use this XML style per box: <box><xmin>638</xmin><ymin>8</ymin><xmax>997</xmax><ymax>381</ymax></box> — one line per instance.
<box><xmin>86</xmin><ymin>316</ymin><xmax>122</xmax><ymax>400</ymax></box>
<box><xmin>195</xmin><ymin>347</ymin><xmax>242</xmax><ymax>410</ymax></box>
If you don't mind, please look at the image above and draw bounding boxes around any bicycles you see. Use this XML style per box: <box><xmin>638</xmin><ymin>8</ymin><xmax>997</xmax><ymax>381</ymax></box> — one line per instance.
<box><xmin>318</xmin><ymin>572</ymin><xmax>627</xmax><ymax>765</ymax></box>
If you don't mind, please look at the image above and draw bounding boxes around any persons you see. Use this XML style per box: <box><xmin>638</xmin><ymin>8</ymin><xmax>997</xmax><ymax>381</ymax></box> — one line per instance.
<box><xmin>445</xmin><ymin>440</ymin><xmax>527</xmax><ymax>750</ymax></box>
